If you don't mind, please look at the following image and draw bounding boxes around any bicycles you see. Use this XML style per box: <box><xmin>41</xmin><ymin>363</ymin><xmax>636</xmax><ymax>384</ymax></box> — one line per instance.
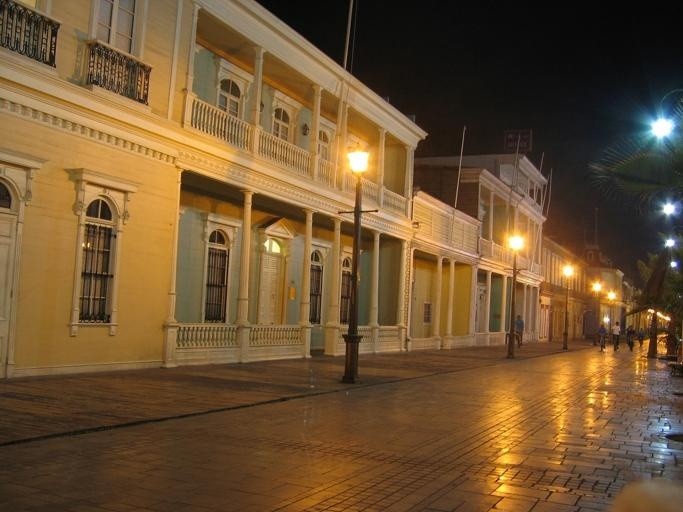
<box><xmin>595</xmin><ymin>331</ymin><xmax>644</xmax><ymax>352</ymax></box>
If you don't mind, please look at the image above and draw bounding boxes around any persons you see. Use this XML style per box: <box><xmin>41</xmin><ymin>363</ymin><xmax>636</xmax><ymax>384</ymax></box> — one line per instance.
<box><xmin>638</xmin><ymin>328</ymin><xmax>645</xmax><ymax>345</ymax></box>
<box><xmin>597</xmin><ymin>323</ymin><xmax>608</xmax><ymax>349</ymax></box>
<box><xmin>611</xmin><ymin>322</ymin><xmax>623</xmax><ymax>351</ymax></box>
<box><xmin>625</xmin><ymin>324</ymin><xmax>635</xmax><ymax>346</ymax></box>
<box><xmin>515</xmin><ymin>314</ymin><xmax>524</xmax><ymax>350</ymax></box>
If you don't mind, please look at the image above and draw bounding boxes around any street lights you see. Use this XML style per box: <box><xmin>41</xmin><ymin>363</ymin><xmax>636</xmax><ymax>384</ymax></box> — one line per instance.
<box><xmin>646</xmin><ymin>307</ymin><xmax>670</xmax><ymax>337</ymax></box>
<box><xmin>607</xmin><ymin>291</ymin><xmax>615</xmax><ymax>335</ymax></box>
<box><xmin>560</xmin><ymin>260</ymin><xmax>574</xmax><ymax>349</ymax></box>
<box><xmin>337</xmin><ymin>148</ymin><xmax>381</xmax><ymax>385</ymax></box>
<box><xmin>592</xmin><ymin>281</ymin><xmax>602</xmax><ymax>343</ymax></box>
<box><xmin>503</xmin><ymin>231</ymin><xmax>528</xmax><ymax>358</ymax></box>
